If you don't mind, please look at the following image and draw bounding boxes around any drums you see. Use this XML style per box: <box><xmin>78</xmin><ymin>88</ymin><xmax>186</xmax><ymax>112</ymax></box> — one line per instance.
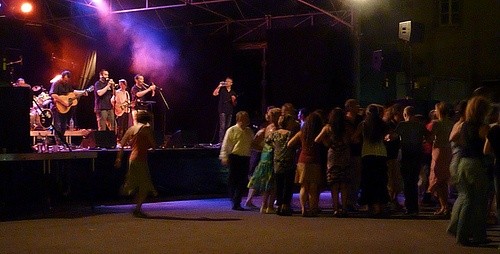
<box><xmin>29</xmin><ymin>107</ymin><xmax>53</xmax><ymax>130</ymax></box>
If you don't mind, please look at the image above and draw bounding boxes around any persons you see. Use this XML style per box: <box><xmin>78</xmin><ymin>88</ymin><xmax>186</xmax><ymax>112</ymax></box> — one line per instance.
<box><xmin>213</xmin><ymin>78</ymin><xmax>239</xmax><ymax>146</ymax></box>
<box><xmin>49</xmin><ymin>70</ymin><xmax>159</xmax><ymax>151</ymax></box>
<box><xmin>217</xmin><ymin>84</ymin><xmax>500</xmax><ymax>246</ymax></box>
<box><xmin>115</xmin><ymin>112</ymin><xmax>158</xmax><ymax>218</ymax></box>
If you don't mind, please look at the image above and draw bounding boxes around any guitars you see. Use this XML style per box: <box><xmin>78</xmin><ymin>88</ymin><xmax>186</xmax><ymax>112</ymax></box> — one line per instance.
<box><xmin>115</xmin><ymin>97</ymin><xmax>146</xmax><ymax>117</ymax></box>
<box><xmin>53</xmin><ymin>85</ymin><xmax>95</xmax><ymax>114</ymax></box>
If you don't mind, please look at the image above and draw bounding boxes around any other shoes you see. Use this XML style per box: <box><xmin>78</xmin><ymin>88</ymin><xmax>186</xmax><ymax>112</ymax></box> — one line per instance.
<box><xmin>260</xmin><ymin>208</ymin><xmax>267</xmax><ymax>213</ymax></box>
<box><xmin>434</xmin><ymin>207</ymin><xmax>450</xmax><ymax>216</ymax></box>
<box><xmin>276</xmin><ymin>208</ymin><xmax>280</xmax><ymax>214</ymax></box>
<box><xmin>244</xmin><ymin>204</ymin><xmax>259</xmax><ymax>210</ymax></box>
<box><xmin>233</xmin><ymin>206</ymin><xmax>244</xmax><ymax>211</ymax></box>
<box><xmin>279</xmin><ymin>209</ymin><xmax>291</xmax><ymax>215</ymax></box>
<box><xmin>266</xmin><ymin>208</ymin><xmax>278</xmax><ymax>213</ymax></box>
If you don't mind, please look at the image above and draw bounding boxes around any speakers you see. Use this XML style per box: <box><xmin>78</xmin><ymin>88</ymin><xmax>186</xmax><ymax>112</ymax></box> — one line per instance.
<box><xmin>398</xmin><ymin>19</ymin><xmax>426</xmax><ymax>43</ymax></box>
<box><xmin>80</xmin><ymin>130</ymin><xmax>116</xmax><ymax>150</ymax></box>
<box><xmin>370</xmin><ymin>49</ymin><xmax>402</xmax><ymax>72</ymax></box>
